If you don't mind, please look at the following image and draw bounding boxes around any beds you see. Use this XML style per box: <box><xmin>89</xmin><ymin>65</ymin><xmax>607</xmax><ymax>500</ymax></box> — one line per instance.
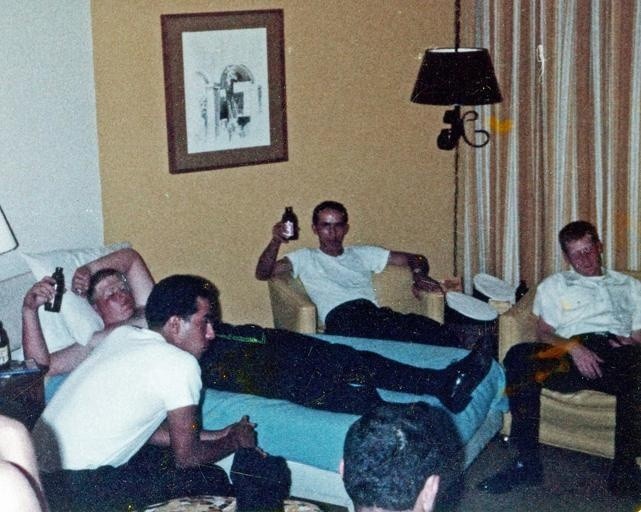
<box><xmin>42</xmin><ymin>322</ymin><xmax>512</xmax><ymax>511</ymax></box>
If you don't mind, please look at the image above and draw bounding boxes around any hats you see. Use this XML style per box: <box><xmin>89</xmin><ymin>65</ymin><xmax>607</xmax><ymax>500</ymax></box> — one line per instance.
<box><xmin>445</xmin><ymin>273</ymin><xmax>515</xmax><ymax>325</ymax></box>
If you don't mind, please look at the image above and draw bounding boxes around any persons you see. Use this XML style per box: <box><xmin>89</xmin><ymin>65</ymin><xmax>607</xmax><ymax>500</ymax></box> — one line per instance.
<box><xmin>22</xmin><ymin>248</ymin><xmax>494</xmax><ymax>416</ymax></box>
<box><xmin>476</xmin><ymin>219</ymin><xmax>641</xmax><ymax>502</ymax></box>
<box><xmin>30</xmin><ymin>273</ymin><xmax>257</xmax><ymax>511</ymax></box>
<box><xmin>255</xmin><ymin>200</ymin><xmax>463</xmax><ymax>348</ymax></box>
<box><xmin>339</xmin><ymin>400</ymin><xmax>466</xmax><ymax>512</ymax></box>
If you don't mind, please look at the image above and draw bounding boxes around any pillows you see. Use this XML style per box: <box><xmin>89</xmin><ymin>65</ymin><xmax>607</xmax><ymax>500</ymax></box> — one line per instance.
<box><xmin>22</xmin><ymin>241</ymin><xmax>134</xmax><ymax>350</ymax></box>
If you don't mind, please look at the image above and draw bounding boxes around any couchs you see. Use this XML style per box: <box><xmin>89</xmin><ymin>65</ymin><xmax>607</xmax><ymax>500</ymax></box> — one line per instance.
<box><xmin>496</xmin><ymin>267</ymin><xmax>641</xmax><ymax>470</ymax></box>
<box><xmin>271</xmin><ymin>260</ymin><xmax>446</xmax><ymax>334</ymax></box>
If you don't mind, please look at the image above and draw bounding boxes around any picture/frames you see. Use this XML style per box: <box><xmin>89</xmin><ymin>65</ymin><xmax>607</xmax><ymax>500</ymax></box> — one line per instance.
<box><xmin>159</xmin><ymin>9</ymin><xmax>290</xmax><ymax>176</ymax></box>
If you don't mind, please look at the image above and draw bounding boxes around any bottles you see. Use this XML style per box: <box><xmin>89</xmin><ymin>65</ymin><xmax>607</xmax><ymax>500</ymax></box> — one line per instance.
<box><xmin>43</xmin><ymin>266</ymin><xmax>65</xmax><ymax>312</ymax></box>
<box><xmin>516</xmin><ymin>280</ymin><xmax>527</xmax><ymax>302</ymax></box>
<box><xmin>279</xmin><ymin>206</ymin><xmax>300</xmax><ymax>242</ymax></box>
<box><xmin>0</xmin><ymin>322</ymin><xmax>12</xmax><ymax>369</ymax></box>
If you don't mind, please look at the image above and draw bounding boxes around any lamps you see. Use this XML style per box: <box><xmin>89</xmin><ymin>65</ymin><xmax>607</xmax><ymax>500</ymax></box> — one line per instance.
<box><xmin>409</xmin><ymin>2</ymin><xmax>503</xmax><ymax>149</ymax></box>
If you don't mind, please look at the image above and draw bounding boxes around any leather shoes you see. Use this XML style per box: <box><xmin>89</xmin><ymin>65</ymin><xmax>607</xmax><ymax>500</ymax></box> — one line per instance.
<box><xmin>607</xmin><ymin>462</ymin><xmax>641</xmax><ymax>498</ymax></box>
<box><xmin>436</xmin><ymin>335</ymin><xmax>493</xmax><ymax>414</ymax></box>
<box><xmin>477</xmin><ymin>459</ymin><xmax>545</xmax><ymax>494</ymax></box>
<box><xmin>229</xmin><ymin>447</ymin><xmax>292</xmax><ymax>512</ymax></box>
